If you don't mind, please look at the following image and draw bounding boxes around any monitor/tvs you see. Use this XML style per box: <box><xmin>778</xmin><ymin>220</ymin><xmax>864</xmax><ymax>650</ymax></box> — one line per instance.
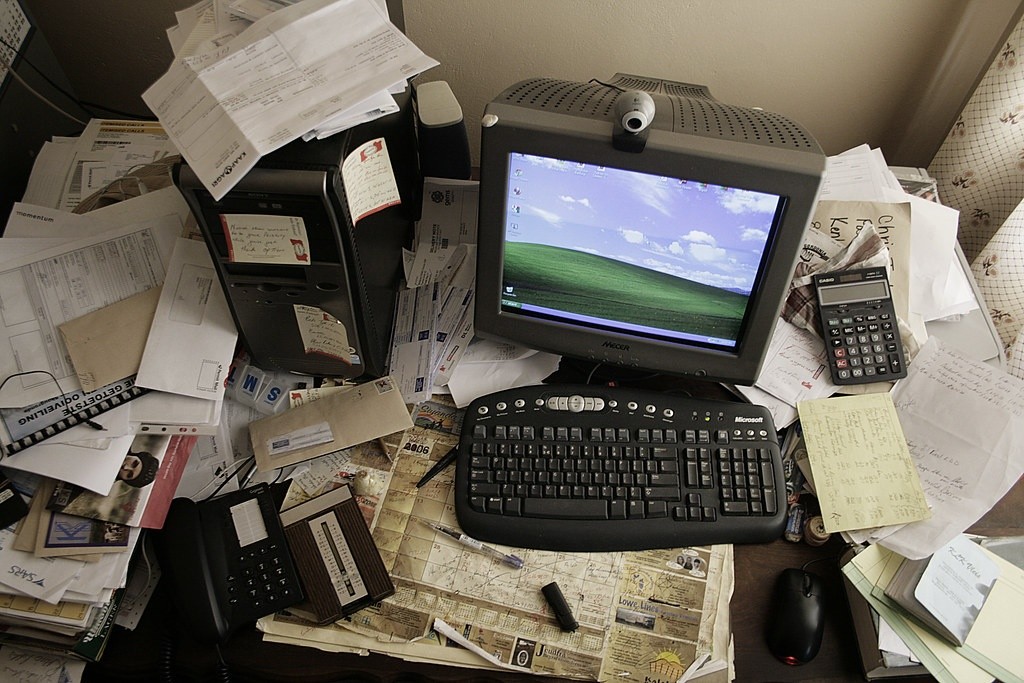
<box><xmin>475</xmin><ymin>71</ymin><xmax>826</xmax><ymax>399</ymax></box>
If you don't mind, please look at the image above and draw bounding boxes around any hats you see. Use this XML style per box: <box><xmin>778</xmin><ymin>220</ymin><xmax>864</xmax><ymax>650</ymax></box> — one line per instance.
<box><xmin>124</xmin><ymin>452</ymin><xmax>159</xmax><ymax>488</ymax></box>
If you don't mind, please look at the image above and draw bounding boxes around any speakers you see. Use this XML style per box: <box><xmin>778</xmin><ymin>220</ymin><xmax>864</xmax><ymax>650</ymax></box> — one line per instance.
<box><xmin>417</xmin><ymin>80</ymin><xmax>472</xmax><ymax>181</ymax></box>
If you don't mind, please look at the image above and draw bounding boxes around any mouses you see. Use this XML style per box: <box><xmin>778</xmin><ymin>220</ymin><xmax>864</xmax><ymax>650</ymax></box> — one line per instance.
<box><xmin>762</xmin><ymin>568</ymin><xmax>825</xmax><ymax>665</ymax></box>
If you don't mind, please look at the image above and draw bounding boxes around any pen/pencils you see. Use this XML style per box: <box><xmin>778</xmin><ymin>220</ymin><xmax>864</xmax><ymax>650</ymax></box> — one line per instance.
<box><xmin>239</xmin><ymin>460</ymin><xmax>257</xmax><ymax>489</ymax></box>
<box><xmin>408</xmin><ymin>515</ymin><xmax>525</xmax><ymax>570</ymax></box>
<box><xmin>414</xmin><ymin>443</ymin><xmax>458</xmax><ymax>491</ymax></box>
<box><xmin>378</xmin><ymin>437</ymin><xmax>393</xmax><ymax>463</ymax></box>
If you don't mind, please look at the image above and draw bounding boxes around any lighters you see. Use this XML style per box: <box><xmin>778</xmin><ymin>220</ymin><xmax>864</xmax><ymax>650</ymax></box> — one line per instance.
<box><xmin>541</xmin><ymin>582</ymin><xmax>580</xmax><ymax>634</ymax></box>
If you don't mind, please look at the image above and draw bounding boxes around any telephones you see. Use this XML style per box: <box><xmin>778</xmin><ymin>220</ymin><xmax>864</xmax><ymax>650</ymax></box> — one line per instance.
<box><xmin>160</xmin><ymin>480</ymin><xmax>305</xmax><ymax>651</ymax></box>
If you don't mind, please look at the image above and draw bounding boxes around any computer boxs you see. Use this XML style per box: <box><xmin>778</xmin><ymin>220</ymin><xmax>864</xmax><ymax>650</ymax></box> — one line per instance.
<box><xmin>169</xmin><ymin>1</ymin><xmax>422</xmax><ymax>382</ymax></box>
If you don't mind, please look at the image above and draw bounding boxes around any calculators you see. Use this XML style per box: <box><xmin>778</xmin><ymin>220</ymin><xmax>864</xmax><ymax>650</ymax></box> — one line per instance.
<box><xmin>813</xmin><ymin>265</ymin><xmax>909</xmax><ymax>386</ymax></box>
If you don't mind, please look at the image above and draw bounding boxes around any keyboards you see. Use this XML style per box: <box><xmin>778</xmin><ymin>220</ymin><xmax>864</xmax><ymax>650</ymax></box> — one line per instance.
<box><xmin>454</xmin><ymin>382</ymin><xmax>788</xmax><ymax>552</ymax></box>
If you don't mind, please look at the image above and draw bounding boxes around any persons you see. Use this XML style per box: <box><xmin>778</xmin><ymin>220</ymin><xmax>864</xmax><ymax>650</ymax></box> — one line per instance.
<box><xmin>677</xmin><ymin>556</ymin><xmax>684</xmax><ymax>565</ymax></box>
<box><xmin>684</xmin><ymin>557</ymin><xmax>693</xmax><ymax>569</ymax></box>
<box><xmin>115</xmin><ymin>448</ymin><xmax>160</xmax><ymax>488</ymax></box>
<box><xmin>694</xmin><ymin>559</ymin><xmax>701</xmax><ymax>568</ymax></box>
